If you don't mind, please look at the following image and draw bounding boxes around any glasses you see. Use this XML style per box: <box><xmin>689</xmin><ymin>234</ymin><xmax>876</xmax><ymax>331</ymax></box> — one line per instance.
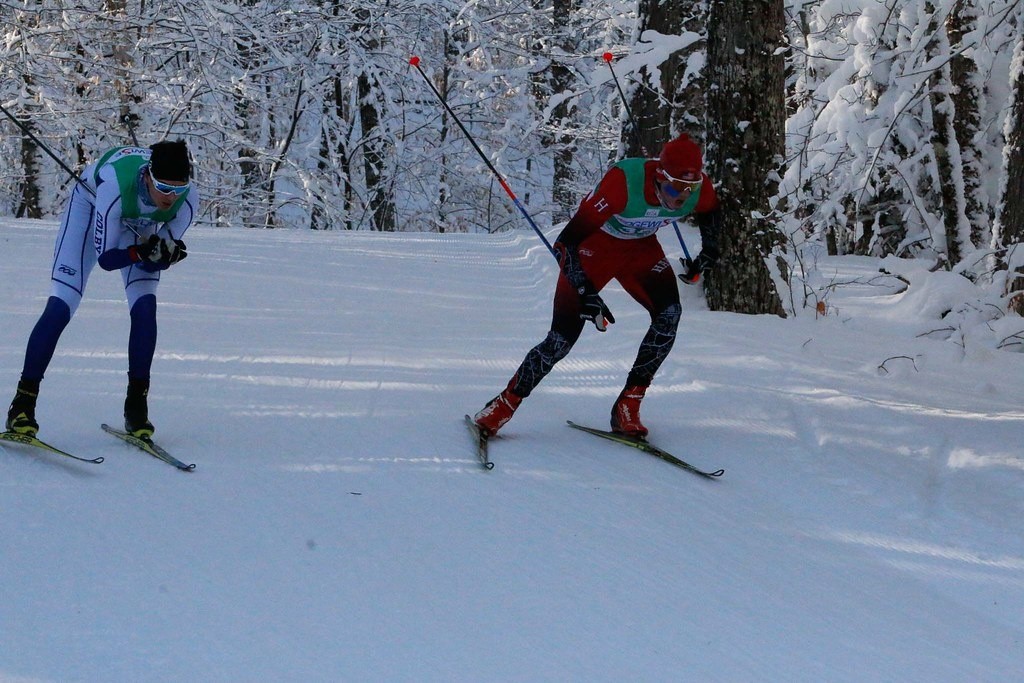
<box><xmin>661</xmin><ymin>173</ymin><xmax>703</xmax><ymax>192</ymax></box>
<box><xmin>148</xmin><ymin>167</ymin><xmax>190</xmax><ymax>196</ymax></box>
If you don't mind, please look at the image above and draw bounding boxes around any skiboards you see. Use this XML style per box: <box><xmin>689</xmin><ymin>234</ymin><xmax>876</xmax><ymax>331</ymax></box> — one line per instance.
<box><xmin>465</xmin><ymin>414</ymin><xmax>725</xmax><ymax>477</ymax></box>
<box><xmin>0</xmin><ymin>421</ymin><xmax>198</xmax><ymax>472</ymax></box>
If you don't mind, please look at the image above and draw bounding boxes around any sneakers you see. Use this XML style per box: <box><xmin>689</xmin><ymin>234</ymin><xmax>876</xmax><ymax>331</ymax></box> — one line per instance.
<box><xmin>124</xmin><ymin>410</ymin><xmax>154</xmax><ymax>439</ymax></box>
<box><xmin>611</xmin><ymin>381</ymin><xmax>648</xmax><ymax>439</ymax></box>
<box><xmin>474</xmin><ymin>389</ymin><xmax>522</xmax><ymax>437</ymax></box>
<box><xmin>5</xmin><ymin>381</ymin><xmax>40</xmax><ymax>436</ymax></box>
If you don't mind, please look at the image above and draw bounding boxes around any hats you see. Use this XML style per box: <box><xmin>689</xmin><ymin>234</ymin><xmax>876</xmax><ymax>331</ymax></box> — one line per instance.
<box><xmin>656</xmin><ymin>133</ymin><xmax>702</xmax><ymax>183</ymax></box>
<box><xmin>149</xmin><ymin>137</ymin><xmax>194</xmax><ymax>182</ymax></box>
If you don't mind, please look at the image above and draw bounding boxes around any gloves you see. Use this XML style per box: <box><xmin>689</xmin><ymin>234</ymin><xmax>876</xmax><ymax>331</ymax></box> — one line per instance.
<box><xmin>127</xmin><ymin>234</ymin><xmax>187</xmax><ymax>274</ymax></box>
<box><xmin>678</xmin><ymin>253</ymin><xmax>714</xmax><ymax>286</ymax></box>
<box><xmin>573</xmin><ymin>279</ymin><xmax>615</xmax><ymax>332</ymax></box>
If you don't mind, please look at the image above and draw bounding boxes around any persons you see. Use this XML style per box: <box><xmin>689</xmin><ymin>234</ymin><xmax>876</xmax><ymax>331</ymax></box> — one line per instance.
<box><xmin>6</xmin><ymin>138</ymin><xmax>199</xmax><ymax>437</ymax></box>
<box><xmin>473</xmin><ymin>133</ymin><xmax>722</xmax><ymax>439</ymax></box>
